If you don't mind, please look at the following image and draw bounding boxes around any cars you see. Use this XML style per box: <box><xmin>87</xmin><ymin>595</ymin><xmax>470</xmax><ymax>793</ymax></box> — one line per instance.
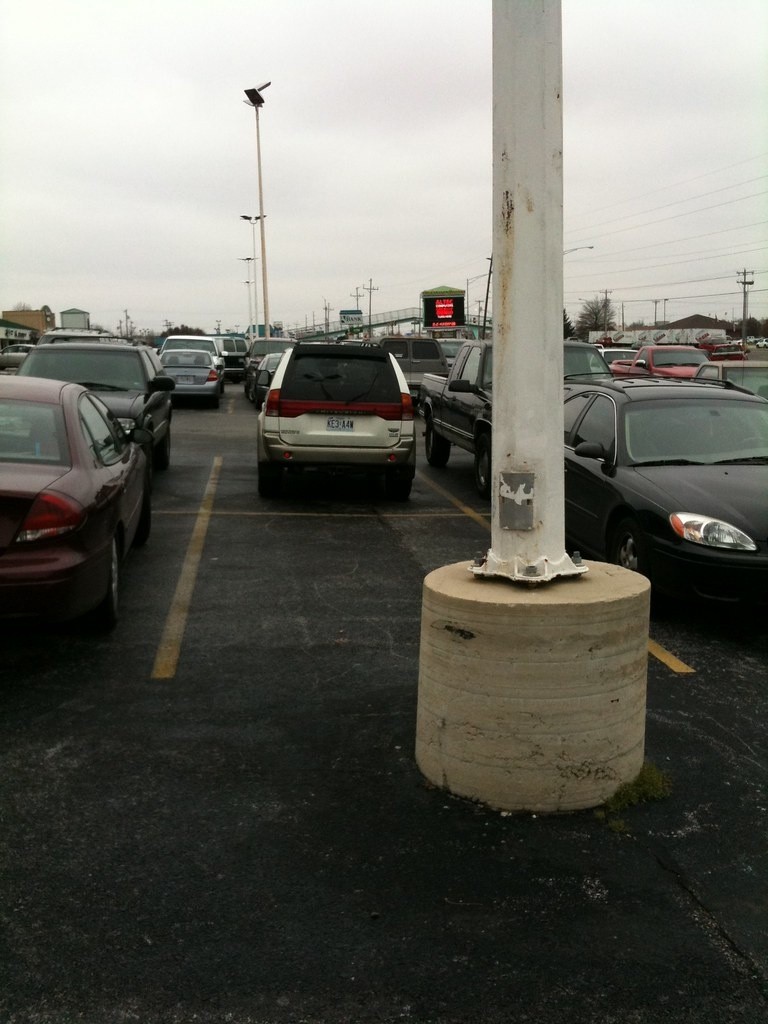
<box><xmin>587</xmin><ymin>346</ymin><xmax>642</xmax><ymax>375</ymax></box>
<box><xmin>14</xmin><ymin>340</ymin><xmax>175</xmax><ymax>471</ymax></box>
<box><xmin>0</xmin><ymin>372</ymin><xmax>155</xmax><ymax>637</ymax></box>
<box><xmin>563</xmin><ymin>377</ymin><xmax>768</xmax><ymax>610</ymax></box>
<box><xmin>0</xmin><ymin>328</ymin><xmax>476</xmax><ymax>413</ymax></box>
<box><xmin>253</xmin><ymin>336</ymin><xmax>420</xmax><ymax>504</ymax></box>
<box><xmin>691</xmin><ymin>357</ymin><xmax>768</xmax><ymax>402</ymax></box>
<box><xmin>710</xmin><ymin>334</ymin><xmax>768</xmax><ymax>360</ymax></box>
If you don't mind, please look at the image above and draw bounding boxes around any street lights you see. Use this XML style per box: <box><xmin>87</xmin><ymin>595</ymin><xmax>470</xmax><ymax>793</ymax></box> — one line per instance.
<box><xmin>244</xmin><ymin>82</ymin><xmax>275</xmax><ymax>339</ymax></box>
<box><xmin>241</xmin><ymin>214</ymin><xmax>269</xmax><ymax>341</ymax></box>
<box><xmin>239</xmin><ymin>257</ymin><xmax>261</xmax><ymax>338</ymax></box>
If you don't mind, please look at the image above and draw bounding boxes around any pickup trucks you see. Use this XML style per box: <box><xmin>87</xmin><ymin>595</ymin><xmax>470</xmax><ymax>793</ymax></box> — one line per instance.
<box><xmin>420</xmin><ymin>335</ymin><xmax>620</xmax><ymax>500</ymax></box>
<box><xmin>611</xmin><ymin>343</ymin><xmax>718</xmax><ymax>379</ymax></box>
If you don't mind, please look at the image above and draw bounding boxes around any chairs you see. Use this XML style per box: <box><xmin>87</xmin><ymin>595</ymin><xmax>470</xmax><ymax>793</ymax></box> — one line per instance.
<box><xmin>168</xmin><ymin>356</ymin><xmax>179</xmax><ymax>365</ymax></box>
<box><xmin>194</xmin><ymin>356</ymin><xmax>205</xmax><ymax>365</ymax></box>
